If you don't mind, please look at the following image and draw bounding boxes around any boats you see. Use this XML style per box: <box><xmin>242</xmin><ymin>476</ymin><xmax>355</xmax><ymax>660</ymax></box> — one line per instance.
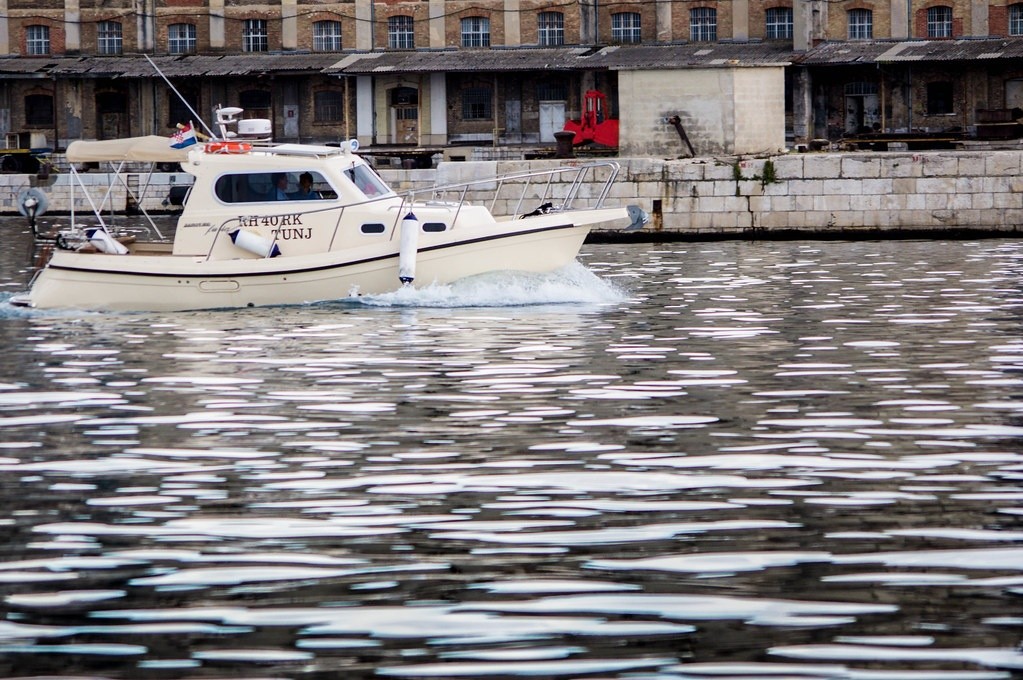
<box><xmin>11</xmin><ymin>52</ymin><xmax>644</xmax><ymax>314</ymax></box>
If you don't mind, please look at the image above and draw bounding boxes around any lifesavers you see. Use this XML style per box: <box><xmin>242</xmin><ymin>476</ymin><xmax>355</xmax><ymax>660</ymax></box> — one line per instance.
<box><xmin>205</xmin><ymin>142</ymin><xmax>252</xmax><ymax>154</ymax></box>
<box><xmin>0</xmin><ymin>154</ymin><xmax>20</xmax><ymax>174</ymax></box>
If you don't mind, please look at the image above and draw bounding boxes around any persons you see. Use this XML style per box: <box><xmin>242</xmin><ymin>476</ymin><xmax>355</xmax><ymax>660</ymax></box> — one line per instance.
<box><xmin>287</xmin><ymin>172</ymin><xmax>321</xmax><ymax>200</ymax></box>
<box><xmin>263</xmin><ymin>174</ymin><xmax>290</xmax><ymax>200</ymax></box>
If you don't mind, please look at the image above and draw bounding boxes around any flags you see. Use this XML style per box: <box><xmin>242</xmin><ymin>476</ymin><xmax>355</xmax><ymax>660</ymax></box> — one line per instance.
<box><xmin>167</xmin><ymin>123</ymin><xmax>198</xmax><ymax>150</ymax></box>
